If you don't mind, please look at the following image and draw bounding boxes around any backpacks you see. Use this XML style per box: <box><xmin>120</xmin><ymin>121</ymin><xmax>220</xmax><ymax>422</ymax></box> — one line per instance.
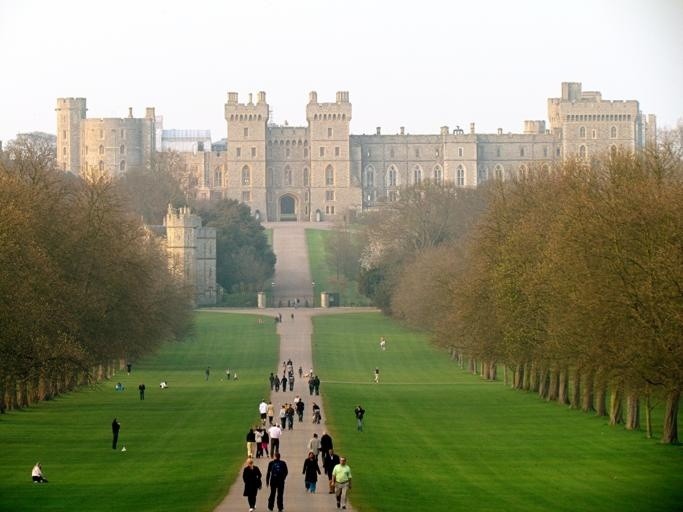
<box><xmin>271</xmin><ymin>460</ymin><xmax>283</xmax><ymax>479</ymax></box>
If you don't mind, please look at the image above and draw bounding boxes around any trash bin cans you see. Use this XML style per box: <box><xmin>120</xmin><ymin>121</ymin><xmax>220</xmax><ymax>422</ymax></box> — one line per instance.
<box><xmin>329</xmin><ymin>293</ymin><xmax>339</xmax><ymax>307</ymax></box>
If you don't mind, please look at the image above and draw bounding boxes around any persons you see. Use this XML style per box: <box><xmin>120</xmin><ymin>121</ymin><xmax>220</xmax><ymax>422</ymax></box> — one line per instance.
<box><xmin>159</xmin><ymin>382</ymin><xmax>168</xmax><ymax>391</ymax></box>
<box><xmin>114</xmin><ymin>382</ymin><xmax>125</xmax><ymax>391</ymax></box>
<box><xmin>138</xmin><ymin>383</ymin><xmax>145</xmax><ymax>400</ymax></box>
<box><xmin>372</xmin><ymin>366</ymin><xmax>379</xmax><ymax>383</ymax></box>
<box><xmin>31</xmin><ymin>463</ymin><xmax>48</xmax><ymax>483</ymax></box>
<box><xmin>203</xmin><ymin>366</ymin><xmax>210</xmax><ymax>382</ymax></box>
<box><xmin>273</xmin><ymin>297</ymin><xmax>310</xmax><ymax>323</ymax></box>
<box><xmin>379</xmin><ymin>336</ymin><xmax>385</xmax><ymax>351</ymax></box>
<box><xmin>111</xmin><ymin>419</ymin><xmax>120</xmax><ymax>449</ymax></box>
<box><xmin>219</xmin><ymin>357</ymin><xmax>352</xmax><ymax>512</ymax></box>
<box><xmin>354</xmin><ymin>407</ymin><xmax>364</xmax><ymax>432</ymax></box>
<box><xmin>126</xmin><ymin>362</ymin><xmax>132</xmax><ymax>376</ymax></box>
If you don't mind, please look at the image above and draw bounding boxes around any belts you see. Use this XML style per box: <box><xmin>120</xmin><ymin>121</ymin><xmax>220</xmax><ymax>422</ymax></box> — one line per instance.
<box><xmin>336</xmin><ymin>481</ymin><xmax>349</xmax><ymax>484</ymax></box>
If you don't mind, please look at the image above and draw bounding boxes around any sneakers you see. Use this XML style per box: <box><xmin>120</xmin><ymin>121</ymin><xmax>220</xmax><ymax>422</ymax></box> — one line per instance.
<box><xmin>247</xmin><ymin>453</ymin><xmax>273</xmax><ymax>459</ymax></box>
<box><xmin>271</xmin><ymin>388</ymin><xmax>293</xmax><ymax>392</ymax></box>
<box><xmin>329</xmin><ymin>491</ymin><xmax>346</xmax><ymax>509</ymax></box>
<box><xmin>248</xmin><ymin>506</ymin><xmax>283</xmax><ymax>512</ymax></box>
<box><xmin>282</xmin><ymin>419</ymin><xmax>303</xmax><ymax>430</ymax></box>
<box><xmin>310</xmin><ymin>391</ymin><xmax>319</xmax><ymax>395</ymax></box>
